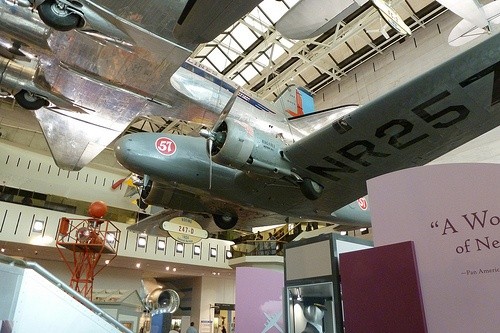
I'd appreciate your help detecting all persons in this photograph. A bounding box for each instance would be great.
[217,325,222,333]
[222,326,226,333]
[237,228,284,255]
[171,325,179,333]
[186,321,197,333]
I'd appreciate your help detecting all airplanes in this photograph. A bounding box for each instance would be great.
[0,0,499,230]
